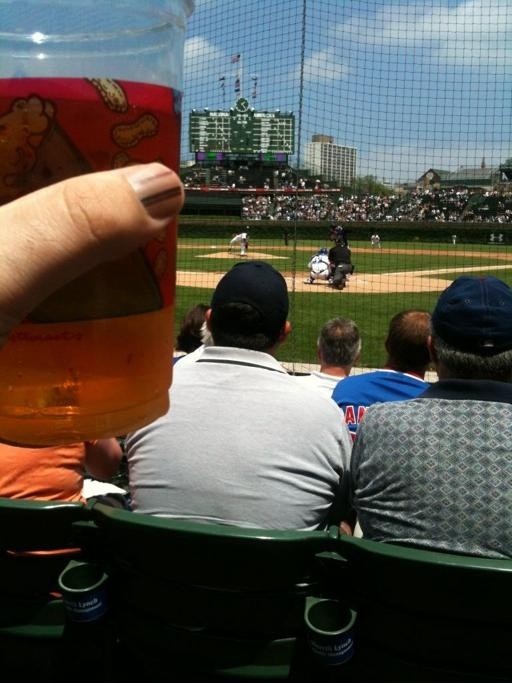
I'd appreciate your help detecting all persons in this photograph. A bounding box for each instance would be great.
[0,435,126,630]
[120,260,357,660]
[328,237,354,290]
[370,231,381,248]
[301,315,362,401]
[351,273,510,558]
[169,302,212,367]
[1,159,188,331]
[327,307,437,437]
[301,246,333,284]
[227,229,250,256]
[328,225,349,249]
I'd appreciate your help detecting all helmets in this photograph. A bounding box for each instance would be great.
[318,246,330,254]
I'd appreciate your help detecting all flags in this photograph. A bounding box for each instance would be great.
[230,53,241,63]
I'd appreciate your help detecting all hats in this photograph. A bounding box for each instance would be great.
[210,257,290,334]
[432,272,511,356]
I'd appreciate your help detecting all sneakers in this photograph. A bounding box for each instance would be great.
[303,278,346,290]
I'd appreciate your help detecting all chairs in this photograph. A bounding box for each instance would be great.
[89,501,337,683]
[331,533,512,682]
[1,496,109,683]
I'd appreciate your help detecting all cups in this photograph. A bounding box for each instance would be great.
[0,0,200,446]
